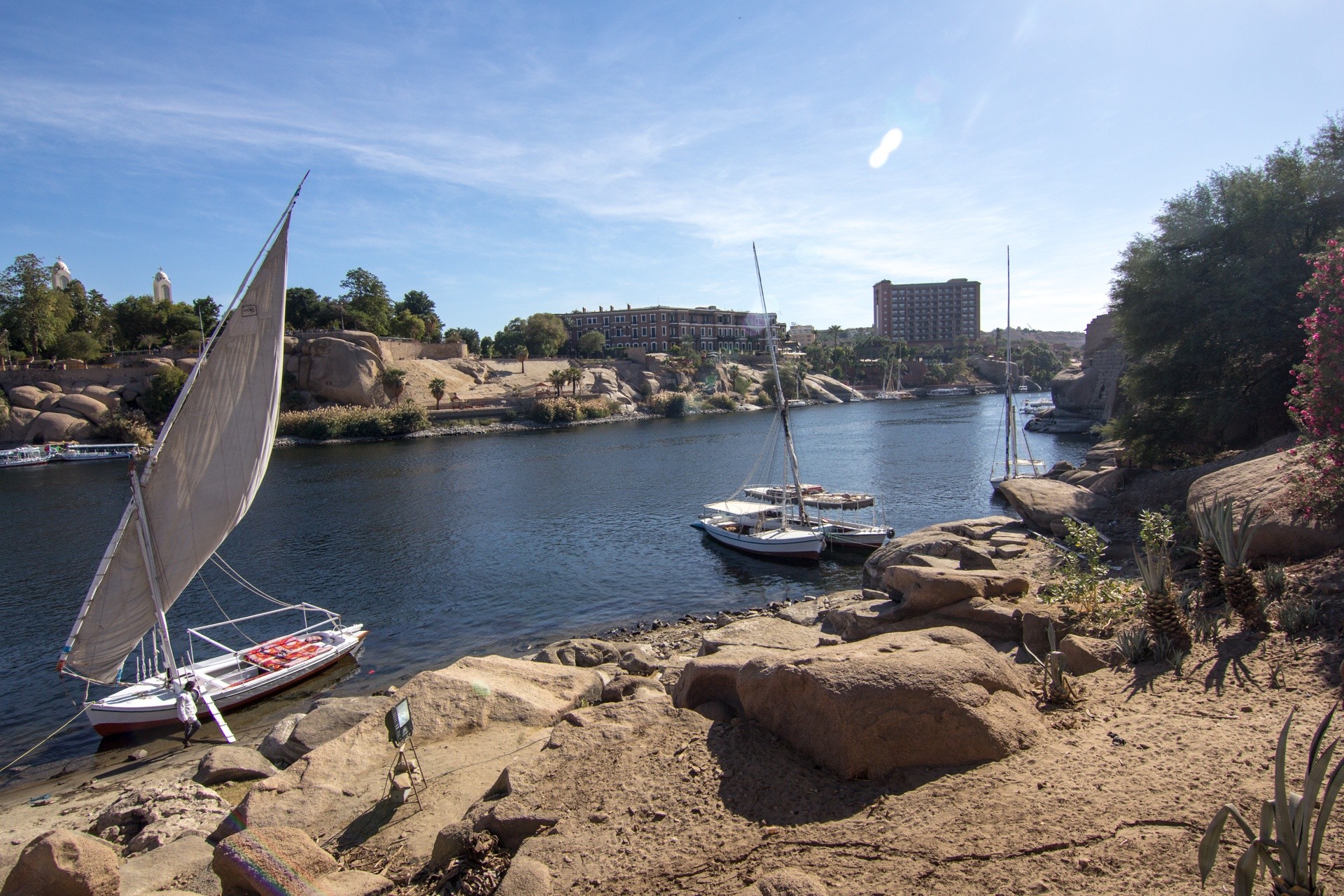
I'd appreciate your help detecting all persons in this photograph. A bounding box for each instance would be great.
[176,681,202,748]
[44,445,49,456]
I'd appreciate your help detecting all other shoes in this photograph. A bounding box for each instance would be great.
[182,740,193,748]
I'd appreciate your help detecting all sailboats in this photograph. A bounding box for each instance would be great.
[988,245,1048,494]
[52,170,369,747]
[693,242,830,568]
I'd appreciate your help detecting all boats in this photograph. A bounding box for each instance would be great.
[0,440,143,468]
[926,387,968,396]
[1019,398,1055,414]
[744,481,895,553]
